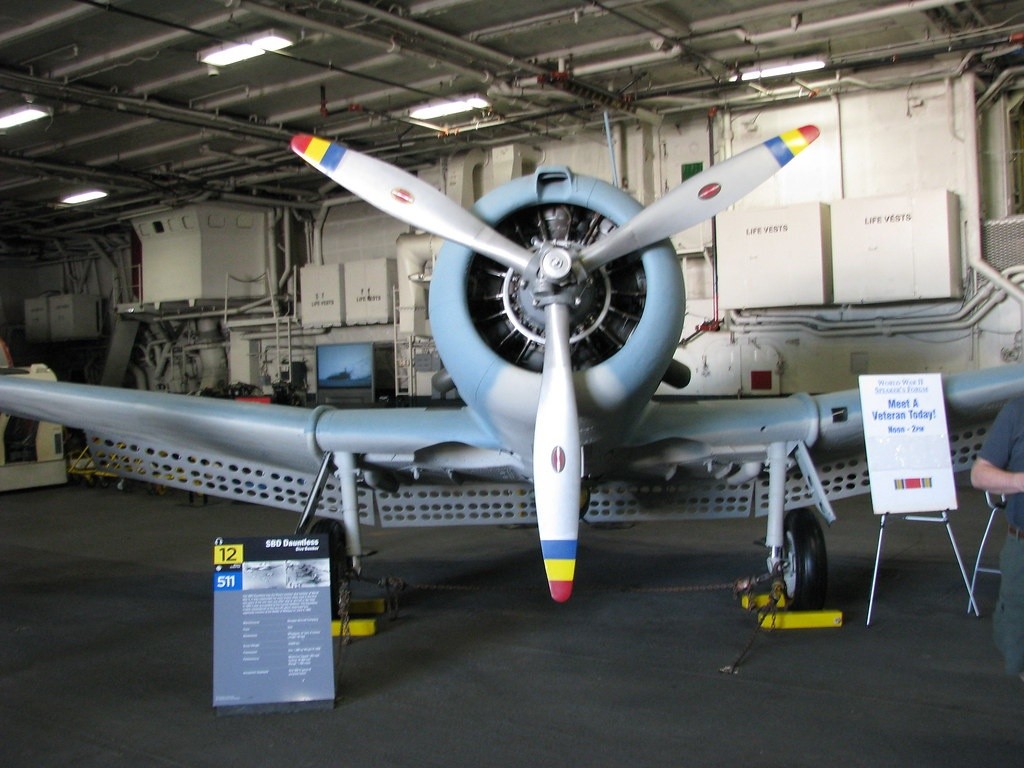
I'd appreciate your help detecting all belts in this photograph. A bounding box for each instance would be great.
[1006,523,1024,542]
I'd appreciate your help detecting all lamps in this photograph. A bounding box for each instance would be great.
[407,91,492,120]
[725,54,828,83]
[0,102,52,130]
[196,27,298,65]
[61,188,107,205]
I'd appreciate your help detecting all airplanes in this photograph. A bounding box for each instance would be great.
[1,132,1022,619]
